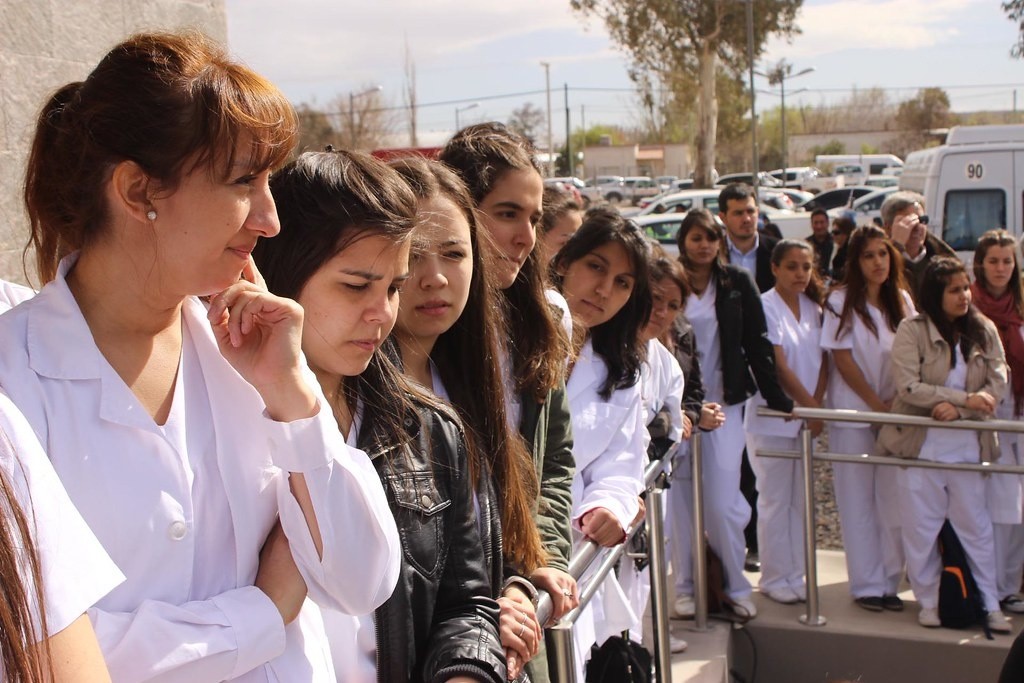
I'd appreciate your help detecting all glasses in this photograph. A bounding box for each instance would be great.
[919,215,929,225]
[831,228,843,235]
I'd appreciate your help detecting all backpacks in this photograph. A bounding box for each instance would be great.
[935,545,994,641]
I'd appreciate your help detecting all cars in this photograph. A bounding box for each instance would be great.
[632,166,902,264]
[544,175,696,202]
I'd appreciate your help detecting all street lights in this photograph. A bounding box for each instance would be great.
[455,102,481,133]
[748,68,814,168]
[541,61,555,177]
[349,86,385,150]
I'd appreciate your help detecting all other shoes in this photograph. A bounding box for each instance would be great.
[741,551,761,572]
[860,596,884,612]
[883,596,904,611]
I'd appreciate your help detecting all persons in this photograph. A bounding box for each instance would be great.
[0,33,403,683]
[0,393,126,683]
[253,122,1024,683]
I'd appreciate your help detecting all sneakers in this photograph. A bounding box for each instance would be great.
[919,607,941,627]
[1002,592,1024,613]
[673,593,697,617]
[987,610,1012,633]
[733,598,758,618]
[669,634,687,653]
[766,585,808,604]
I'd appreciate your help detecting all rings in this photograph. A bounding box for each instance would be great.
[521,610,529,625]
[561,587,574,600]
[518,624,527,637]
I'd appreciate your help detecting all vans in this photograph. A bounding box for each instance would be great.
[815,154,904,175]
[901,125,1024,282]
